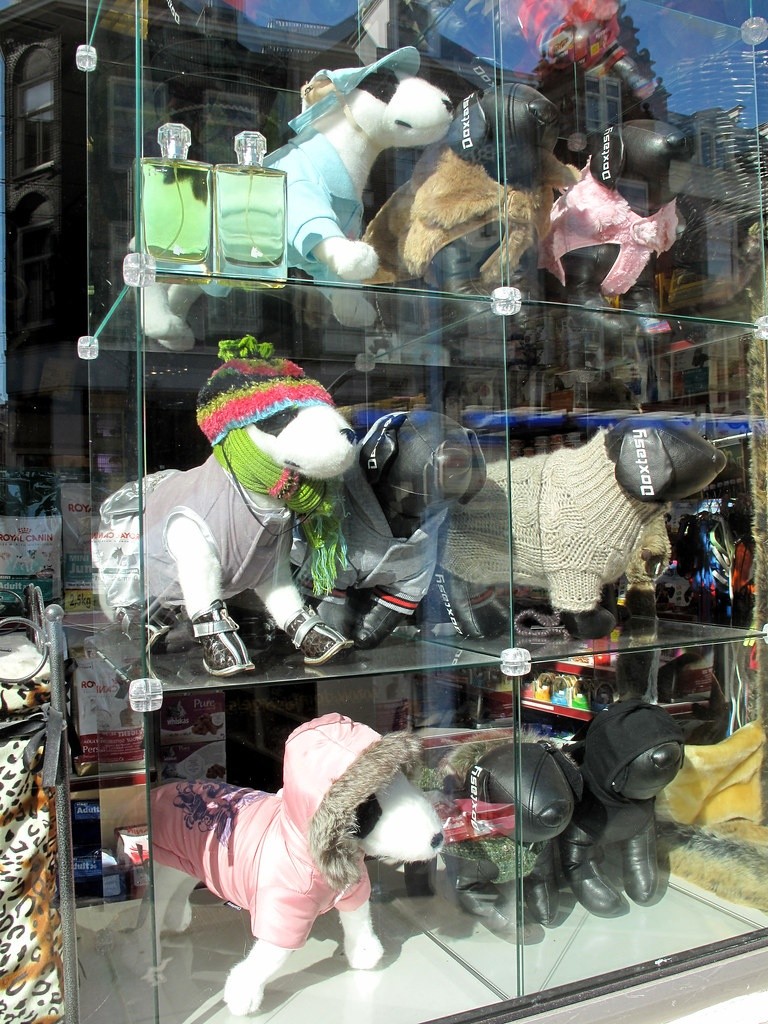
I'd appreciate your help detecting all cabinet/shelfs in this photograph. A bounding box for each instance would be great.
[59,0,768,1024]
[433,294,747,723]
[226,675,419,763]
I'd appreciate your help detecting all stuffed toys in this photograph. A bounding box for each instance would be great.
[122,713,445,1016]
[406,727,583,944]
[128,47,452,351]
[537,697,686,915]
[293,79,564,356]
[232,409,487,647]
[531,120,693,351]
[416,418,727,641]
[87,336,360,677]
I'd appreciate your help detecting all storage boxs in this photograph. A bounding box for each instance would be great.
[153,691,226,747]
[154,739,227,784]
